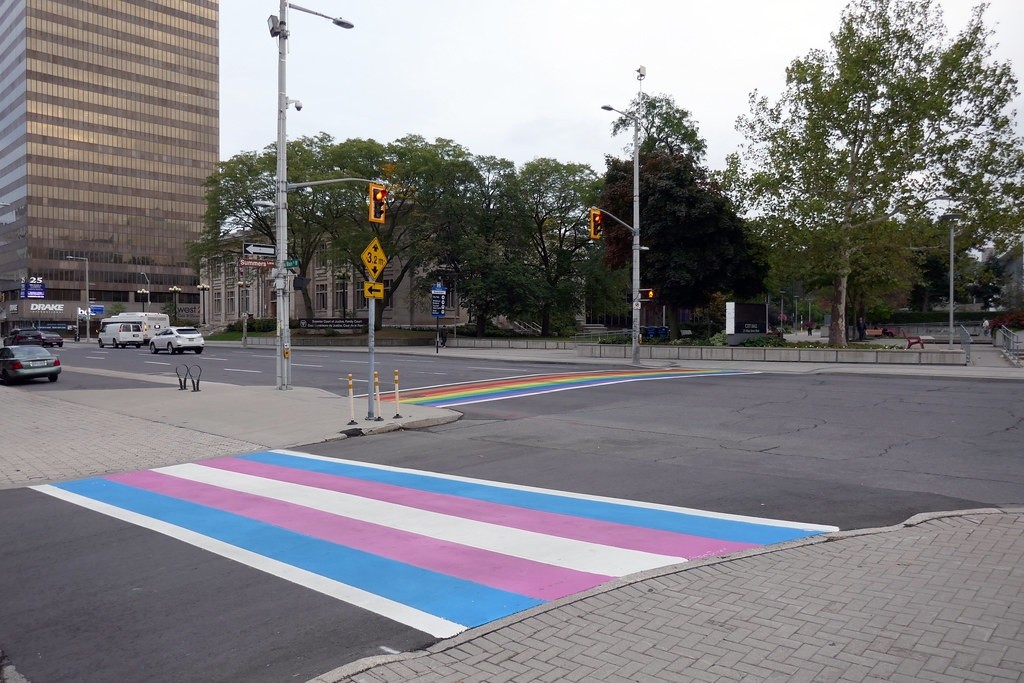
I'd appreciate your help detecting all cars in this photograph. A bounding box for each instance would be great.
[0,345,62,386]
[149,326,206,355]
[2,327,43,346]
[36,330,64,347]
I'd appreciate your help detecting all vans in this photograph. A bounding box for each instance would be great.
[98,322,145,349]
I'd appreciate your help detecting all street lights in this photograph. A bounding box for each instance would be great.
[779,290,786,332]
[266,0,355,394]
[335,267,352,329]
[197,283,211,324]
[794,296,800,335]
[66,255,91,343]
[238,281,251,331]
[943,212,963,351]
[169,285,181,325]
[807,298,813,333]
[601,104,642,366]
[137,289,150,312]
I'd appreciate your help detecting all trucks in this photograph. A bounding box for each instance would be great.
[99,311,171,345]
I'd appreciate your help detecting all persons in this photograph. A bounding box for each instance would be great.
[857,317,867,342]
[806,320,813,336]
[440,325,448,348]
[982,317,990,337]
[880,327,893,336]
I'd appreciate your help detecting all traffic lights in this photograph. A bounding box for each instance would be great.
[369,182,388,224]
[590,208,604,240]
[646,290,654,299]
